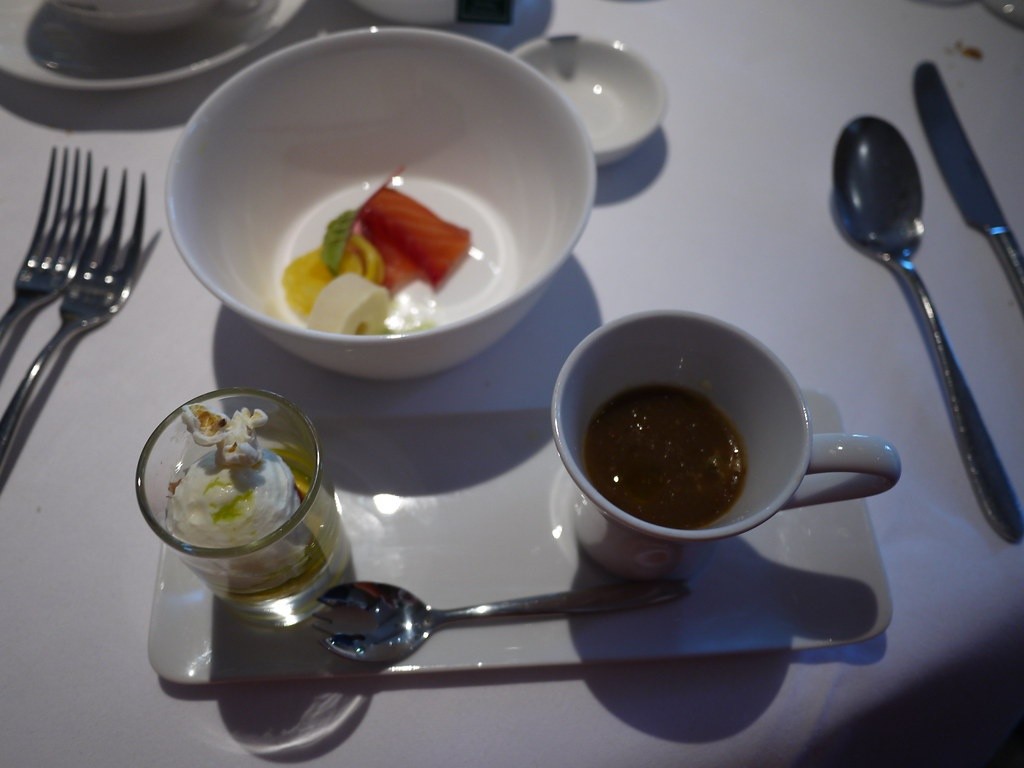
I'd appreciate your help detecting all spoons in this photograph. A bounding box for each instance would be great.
[313,581,687,665]
[831,116,1024,543]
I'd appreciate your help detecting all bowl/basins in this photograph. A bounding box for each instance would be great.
[165,26,595,379]
[48,0,212,35]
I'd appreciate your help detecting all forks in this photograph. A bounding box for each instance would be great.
[0,147,91,359]
[0,167,146,477]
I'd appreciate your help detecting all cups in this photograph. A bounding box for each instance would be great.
[550,309,902,542]
[137,387,345,627]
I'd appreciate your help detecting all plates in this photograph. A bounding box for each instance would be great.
[148,392,894,684]
[513,33,670,166]
[0,0,305,89]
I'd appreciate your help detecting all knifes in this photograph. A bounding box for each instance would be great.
[914,62,1024,318]
